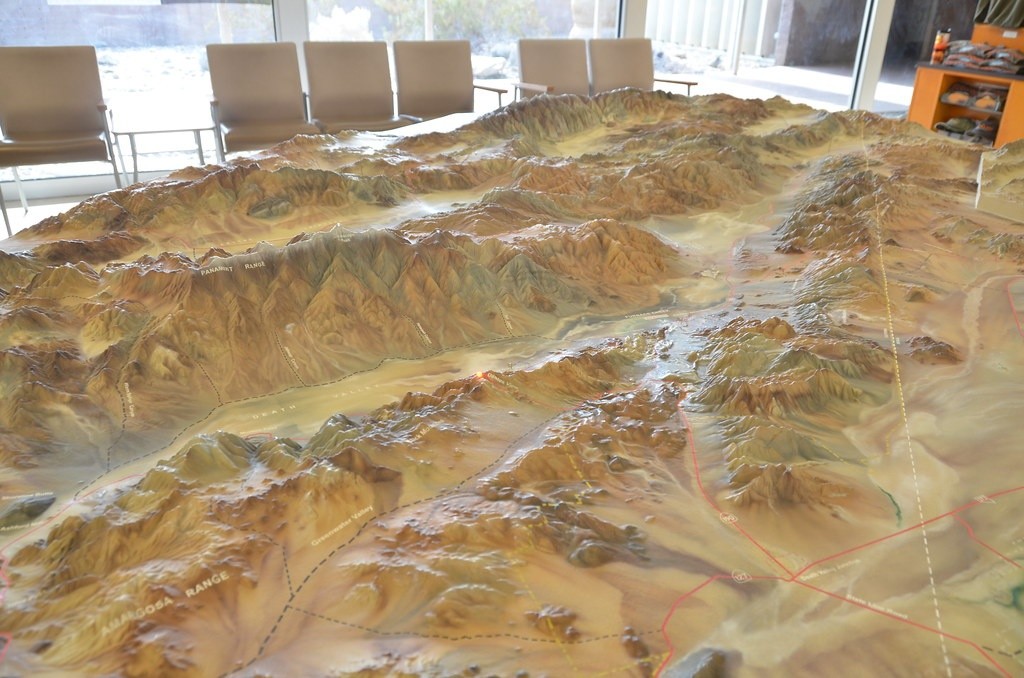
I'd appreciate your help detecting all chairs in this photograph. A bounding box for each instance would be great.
[0,45,121,237]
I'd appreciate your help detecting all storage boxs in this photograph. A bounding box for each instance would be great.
[971,23,1024,51]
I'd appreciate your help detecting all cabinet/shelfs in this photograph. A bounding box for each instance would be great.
[907,61,1024,150]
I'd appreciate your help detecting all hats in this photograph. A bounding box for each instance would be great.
[934,117,975,133]
[967,119,998,141]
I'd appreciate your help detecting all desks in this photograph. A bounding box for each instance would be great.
[114,122,211,184]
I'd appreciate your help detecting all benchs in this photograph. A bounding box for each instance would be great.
[512,39,698,94]
[205,42,507,161]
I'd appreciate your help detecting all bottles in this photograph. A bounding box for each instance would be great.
[930,27,951,65]
